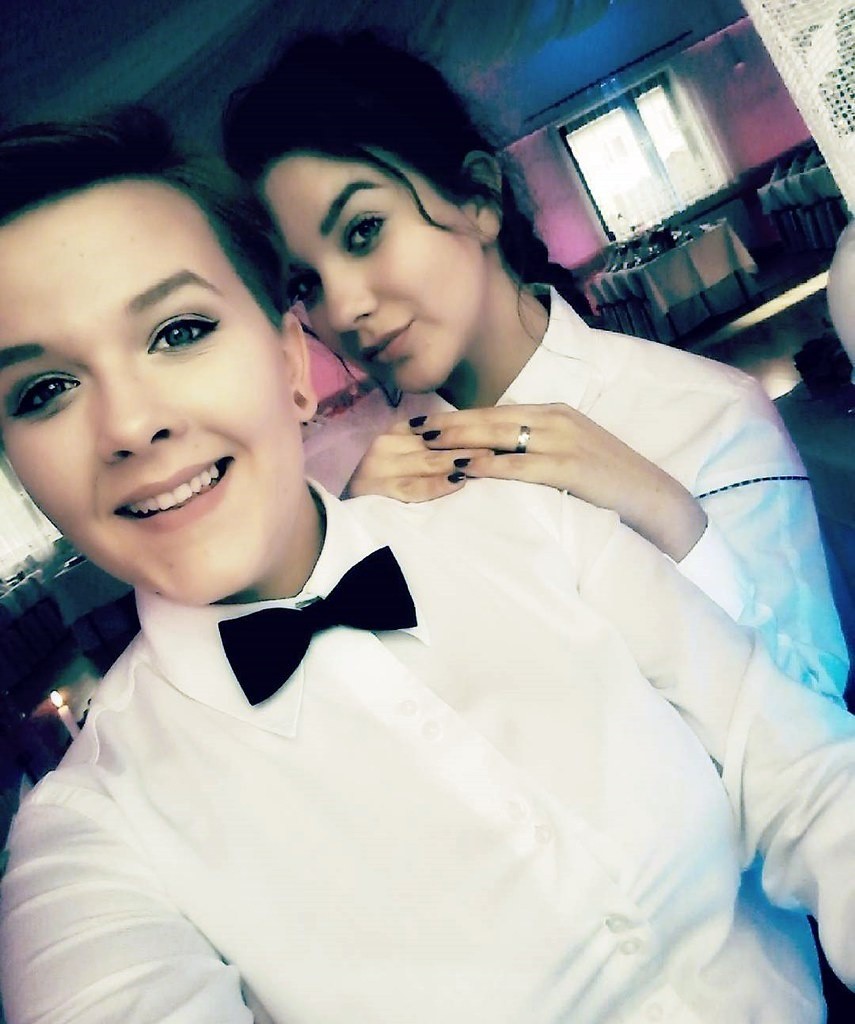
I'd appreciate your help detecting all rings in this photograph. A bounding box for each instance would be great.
[517,425,531,451]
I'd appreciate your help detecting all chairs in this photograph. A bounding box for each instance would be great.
[587,271,662,344]
[756,168,847,251]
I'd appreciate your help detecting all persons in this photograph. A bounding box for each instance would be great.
[0,122,855,1024]
[223,28,849,703]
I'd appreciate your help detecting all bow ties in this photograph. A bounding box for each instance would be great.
[218,546,419,707]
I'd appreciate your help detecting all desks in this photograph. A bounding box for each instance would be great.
[0,569,61,664]
[51,564,135,651]
[583,219,760,342]
